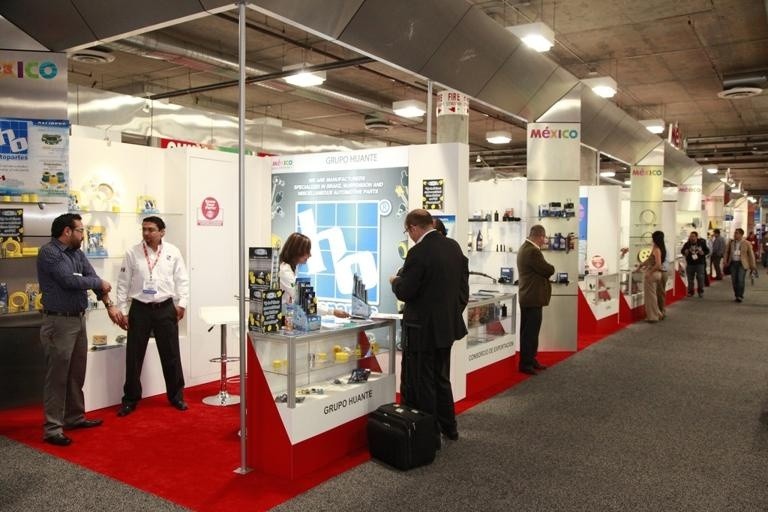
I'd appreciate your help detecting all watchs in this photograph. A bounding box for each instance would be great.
[105,300,112,306]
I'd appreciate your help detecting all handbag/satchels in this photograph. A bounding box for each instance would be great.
[723,266,731,275]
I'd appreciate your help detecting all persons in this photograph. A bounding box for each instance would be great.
[38,213,124,448]
[680,228,768,303]
[635,231,669,316]
[632,232,666,323]
[390,208,471,451]
[517,225,554,375]
[278,233,351,318]
[116,216,190,416]
[431,220,446,238]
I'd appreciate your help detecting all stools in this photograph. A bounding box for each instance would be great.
[198,302,243,408]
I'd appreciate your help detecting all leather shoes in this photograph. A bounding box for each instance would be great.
[46,400,187,445]
[519,363,548,376]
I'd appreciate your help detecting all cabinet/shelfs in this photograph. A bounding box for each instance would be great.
[621,268,649,322]
[578,270,619,335]
[470,217,524,286]
[0,200,73,322]
[72,207,189,352]
[468,289,518,393]
[242,316,399,476]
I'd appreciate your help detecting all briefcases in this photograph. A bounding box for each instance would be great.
[367,403,437,471]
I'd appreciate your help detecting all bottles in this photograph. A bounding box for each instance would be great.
[475,229,483,252]
[494,210,498,221]
[502,304,507,317]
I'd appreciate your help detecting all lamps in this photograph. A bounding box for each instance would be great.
[278,40,327,86]
[637,103,666,134]
[578,57,620,99]
[250,102,282,130]
[388,79,429,120]
[142,99,150,113]
[484,115,514,144]
[498,0,556,52]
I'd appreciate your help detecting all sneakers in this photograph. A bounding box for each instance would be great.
[687,292,743,302]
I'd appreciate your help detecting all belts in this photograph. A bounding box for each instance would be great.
[44,310,85,318]
[132,297,172,311]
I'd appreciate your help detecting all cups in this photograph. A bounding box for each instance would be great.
[20,193,29,202]
[273,360,282,369]
[29,193,38,202]
[3,195,11,202]
[113,206,120,213]
[42,172,65,185]
[81,206,88,211]
[315,352,349,363]
